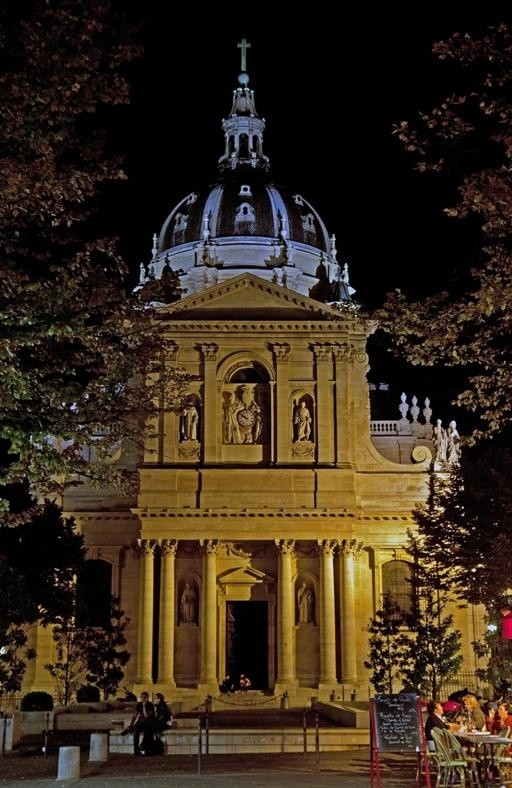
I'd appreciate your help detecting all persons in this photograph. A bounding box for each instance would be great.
[425,700,473,753]
[122,691,155,755]
[223,675,236,696]
[180,400,200,442]
[239,673,252,695]
[458,695,486,732]
[293,401,313,444]
[297,580,313,623]
[484,701,512,779]
[178,581,198,624]
[137,692,174,749]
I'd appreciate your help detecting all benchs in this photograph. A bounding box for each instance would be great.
[110,716,202,736]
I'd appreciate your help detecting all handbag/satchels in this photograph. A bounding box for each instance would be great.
[145,735,164,757]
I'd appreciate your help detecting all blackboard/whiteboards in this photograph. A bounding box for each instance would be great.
[370,693,427,752]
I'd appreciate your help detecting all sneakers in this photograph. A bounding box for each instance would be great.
[121,729,129,736]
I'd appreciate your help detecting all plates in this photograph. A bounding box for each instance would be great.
[472,731,491,736]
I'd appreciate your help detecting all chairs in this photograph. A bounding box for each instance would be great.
[415,718,512,788]
